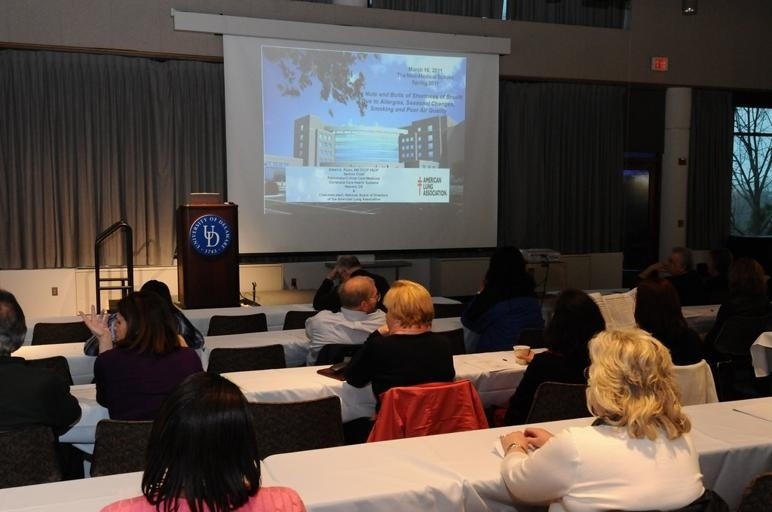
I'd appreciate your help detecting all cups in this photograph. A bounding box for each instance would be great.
[512,344,530,367]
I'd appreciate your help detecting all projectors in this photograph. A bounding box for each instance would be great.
[520,248,561,264]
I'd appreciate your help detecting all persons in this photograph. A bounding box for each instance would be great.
[499,328,708,511]
[77,280,206,421]
[313,255,389,314]
[461,247,544,354]
[486,287,606,428]
[633,247,772,402]
[1,290,81,488]
[305,275,388,366]
[98,371,311,512]
[346,280,455,413]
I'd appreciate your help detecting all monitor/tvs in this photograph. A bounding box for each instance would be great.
[188,191,221,205]
